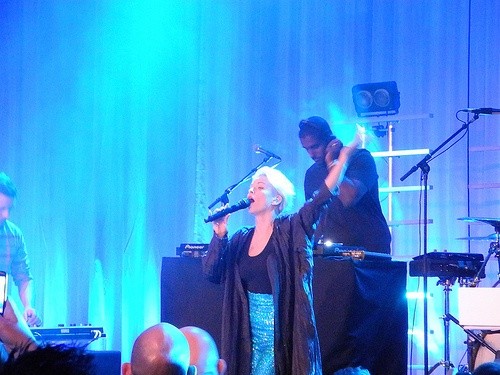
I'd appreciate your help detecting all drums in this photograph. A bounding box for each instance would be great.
[470,332,500,371]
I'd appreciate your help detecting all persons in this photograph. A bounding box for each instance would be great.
[298,116,392,260]
[473,362,500,375]
[202,124,361,375]
[123,322,227,375]
[1,172,41,328]
[0,298,95,375]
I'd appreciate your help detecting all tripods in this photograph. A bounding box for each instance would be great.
[427,279,500,375]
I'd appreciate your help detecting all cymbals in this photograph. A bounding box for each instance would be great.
[454,232,500,242]
[455,217,500,226]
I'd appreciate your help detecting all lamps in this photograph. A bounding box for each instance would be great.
[351,81,400,117]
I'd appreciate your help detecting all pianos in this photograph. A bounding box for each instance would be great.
[29,323,106,350]
[409,251,486,375]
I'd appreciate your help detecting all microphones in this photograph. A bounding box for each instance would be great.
[204,198,251,223]
[253,144,281,160]
[460,108,500,115]
